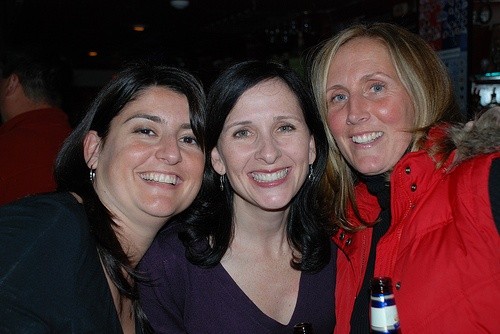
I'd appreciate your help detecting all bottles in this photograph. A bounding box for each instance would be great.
[262,7,313,49]
[369,277,402,334]
[292,322,314,334]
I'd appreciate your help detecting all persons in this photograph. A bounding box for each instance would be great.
[0,64,208,334]
[0,52,74,206]
[307,21,500,333]
[134,58,337,334]
